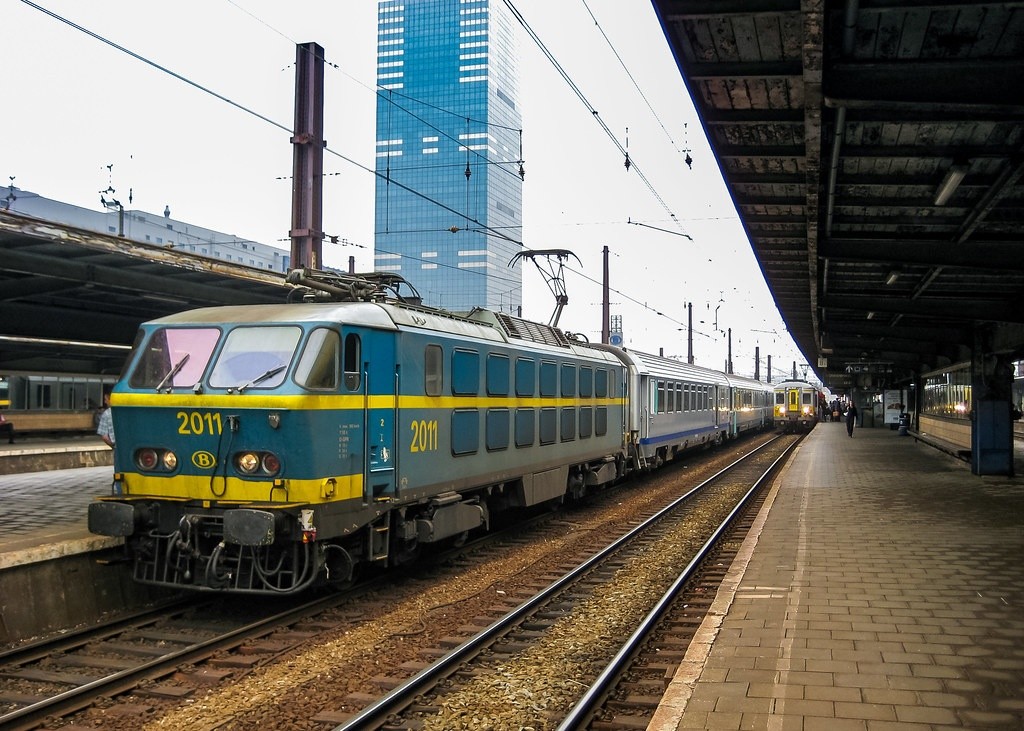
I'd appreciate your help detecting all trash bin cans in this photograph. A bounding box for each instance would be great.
[898,413,911,436]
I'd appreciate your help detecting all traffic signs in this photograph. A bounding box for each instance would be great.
[844,361,896,374]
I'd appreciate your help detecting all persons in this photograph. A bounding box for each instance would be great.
[842,400,859,440]
[821,400,847,422]
[96,389,123,496]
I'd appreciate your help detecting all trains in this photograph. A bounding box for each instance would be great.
[86,265,778,596]
[920,369,971,417]
[773,379,825,433]
[0,370,122,434]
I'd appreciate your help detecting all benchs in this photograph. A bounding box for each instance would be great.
[905,428,972,464]
